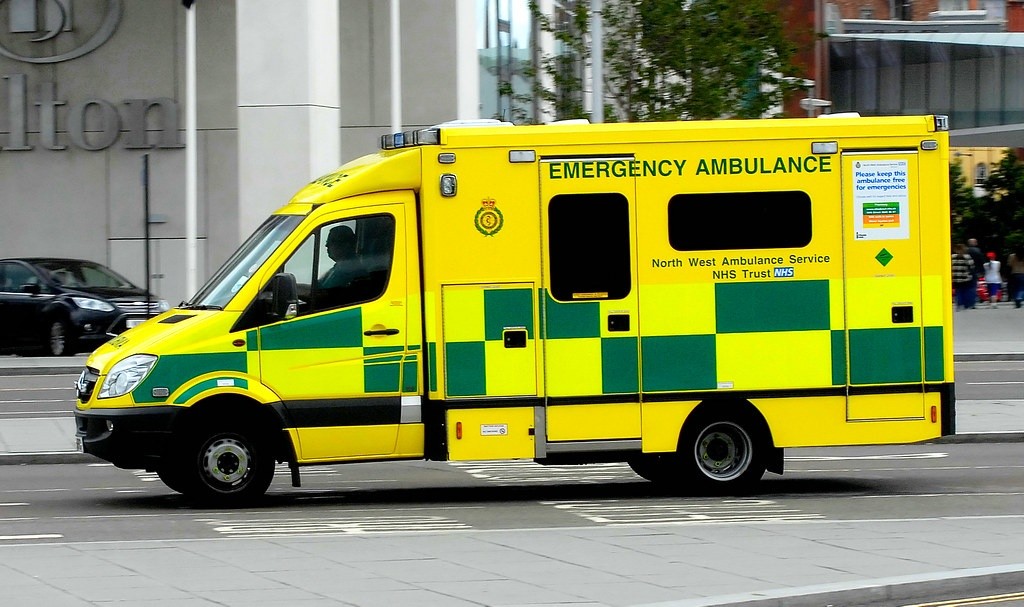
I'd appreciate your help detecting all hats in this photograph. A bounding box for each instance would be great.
[987,252,997,260]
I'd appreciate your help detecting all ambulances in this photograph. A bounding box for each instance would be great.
[72,111,957,512]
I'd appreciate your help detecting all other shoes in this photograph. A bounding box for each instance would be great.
[991,302,998,309]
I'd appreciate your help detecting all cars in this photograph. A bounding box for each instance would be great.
[0,258,171,358]
[976,282,1002,303]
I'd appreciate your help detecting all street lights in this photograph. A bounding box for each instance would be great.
[800,98,831,114]
[783,77,814,118]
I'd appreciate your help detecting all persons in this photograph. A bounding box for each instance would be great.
[951,238,1024,312]
[297,225,371,310]
